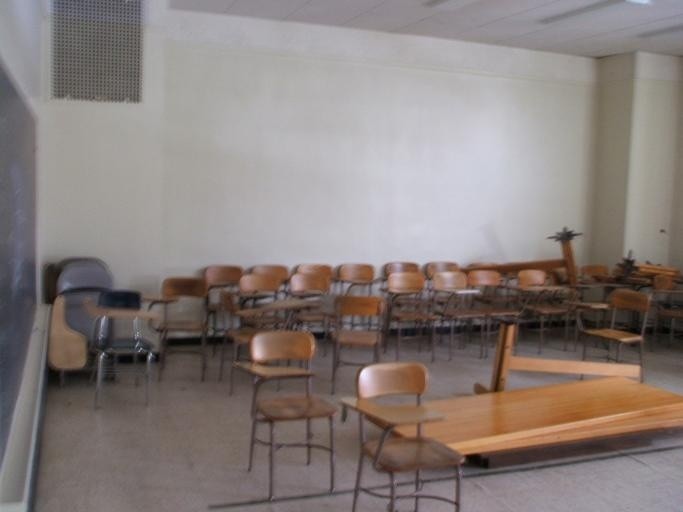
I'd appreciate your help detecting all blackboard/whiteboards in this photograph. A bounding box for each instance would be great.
[0,61,36,466]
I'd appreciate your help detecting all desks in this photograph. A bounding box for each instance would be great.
[367,376,682,469]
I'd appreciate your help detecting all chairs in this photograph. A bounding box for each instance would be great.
[353,361,466,511]
[52,259,681,396]
[247,330,338,501]
[94,288,154,409]
